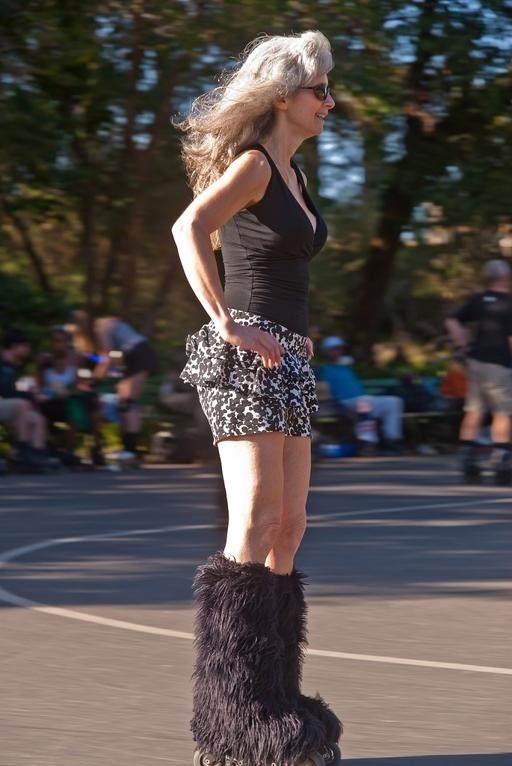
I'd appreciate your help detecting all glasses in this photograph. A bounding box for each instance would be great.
[300,83,333,101]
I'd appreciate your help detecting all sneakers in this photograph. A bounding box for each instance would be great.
[7,445,196,474]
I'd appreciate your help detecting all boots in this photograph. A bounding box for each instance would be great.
[456,439,482,485]
[190,550,327,766]
[489,442,511,485]
[279,565,341,766]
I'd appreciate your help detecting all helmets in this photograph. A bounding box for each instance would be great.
[320,336,345,354]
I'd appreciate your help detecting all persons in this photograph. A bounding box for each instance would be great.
[173,28,342,762]
[311,326,491,455]
[2,306,218,473]
[445,258,511,469]
